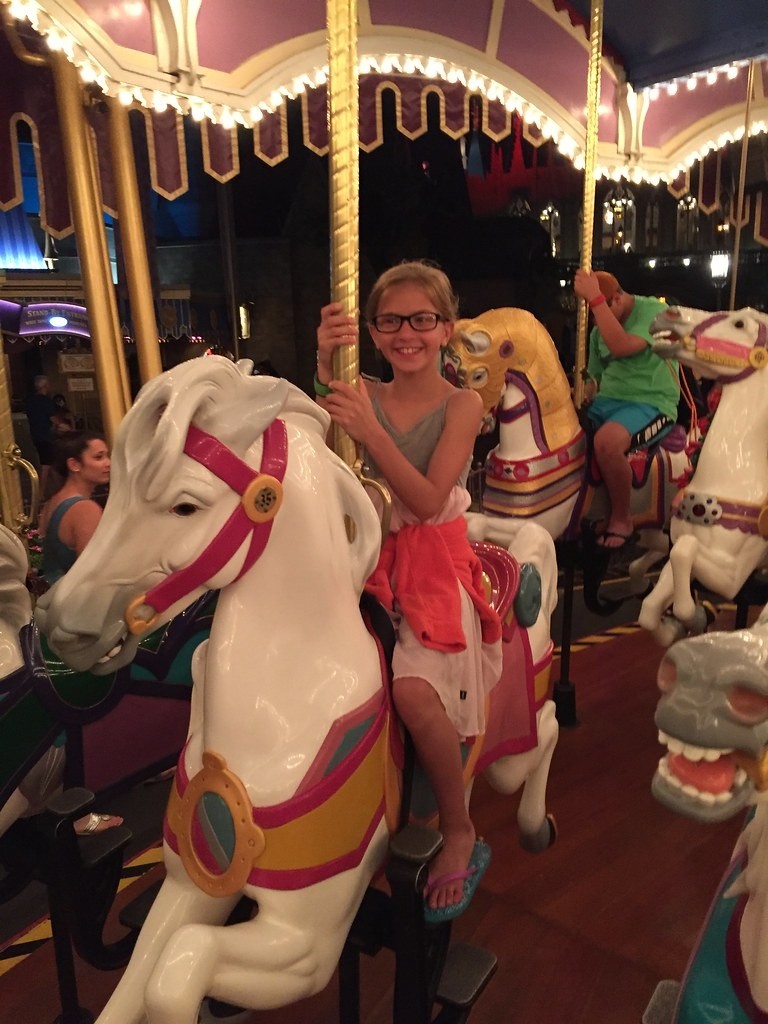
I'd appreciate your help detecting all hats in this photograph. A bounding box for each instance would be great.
[592,271,620,297]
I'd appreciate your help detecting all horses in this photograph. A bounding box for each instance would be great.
[0,303,768,1024]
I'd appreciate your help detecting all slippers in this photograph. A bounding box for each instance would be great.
[420,840,492,922]
[595,530,641,551]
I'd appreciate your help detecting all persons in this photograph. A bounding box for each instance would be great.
[51,393,75,434]
[39,432,109,585]
[573,267,682,554]
[311,263,492,923]
[22,376,54,504]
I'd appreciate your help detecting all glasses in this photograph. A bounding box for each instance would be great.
[369,311,447,334]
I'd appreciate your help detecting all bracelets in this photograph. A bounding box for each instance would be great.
[589,295,604,308]
[312,370,333,397]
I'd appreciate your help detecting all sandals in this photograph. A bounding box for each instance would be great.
[76,813,123,836]
